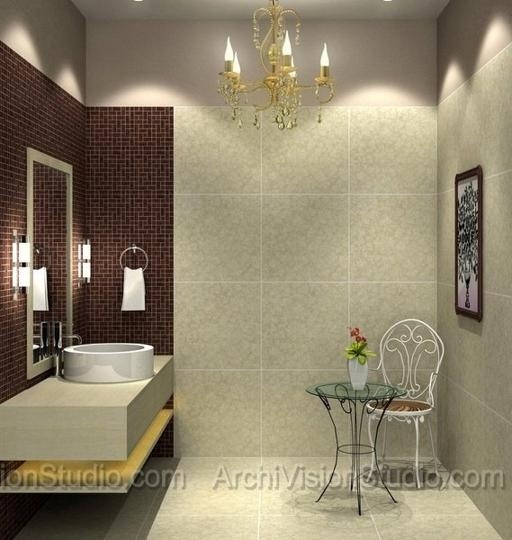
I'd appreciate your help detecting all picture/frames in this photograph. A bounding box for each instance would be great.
[454,166,483,323]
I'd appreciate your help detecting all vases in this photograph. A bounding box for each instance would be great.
[348,359,368,390]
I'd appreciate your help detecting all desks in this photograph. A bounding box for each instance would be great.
[306,382,407,516]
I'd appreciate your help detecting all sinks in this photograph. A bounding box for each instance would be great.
[32,344,40,364]
[63,342,155,384]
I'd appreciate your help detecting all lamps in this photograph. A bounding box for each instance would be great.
[219,0,334,131]
[18,233,31,294]
[82,236,91,285]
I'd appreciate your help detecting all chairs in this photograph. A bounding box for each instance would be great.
[367,318,445,489]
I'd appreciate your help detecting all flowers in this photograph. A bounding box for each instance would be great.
[345,328,377,364]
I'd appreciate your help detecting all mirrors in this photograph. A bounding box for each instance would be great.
[26,146,74,380]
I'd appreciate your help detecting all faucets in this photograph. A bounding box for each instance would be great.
[51,320,83,377]
[33,321,50,360]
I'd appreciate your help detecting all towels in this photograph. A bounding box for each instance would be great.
[33,266,49,312]
[120,267,146,312]
